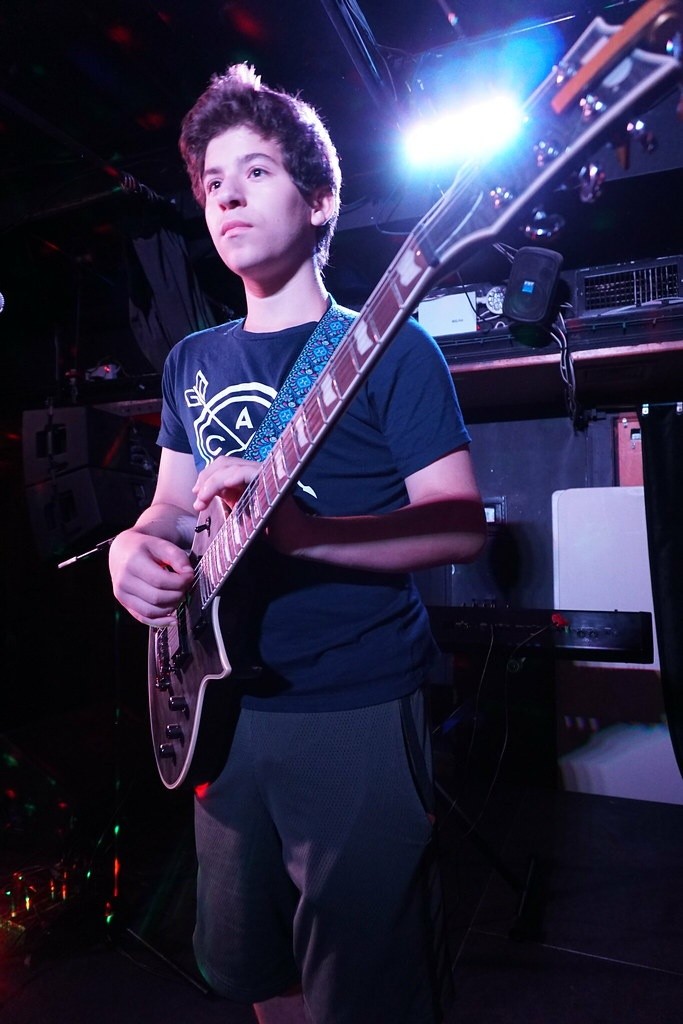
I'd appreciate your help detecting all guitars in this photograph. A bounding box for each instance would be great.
[145,0,683,792]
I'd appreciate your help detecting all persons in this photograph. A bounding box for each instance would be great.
[109,61,486,1024]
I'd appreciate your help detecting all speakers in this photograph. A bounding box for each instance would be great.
[21,407,163,562]
[501,246,564,326]
